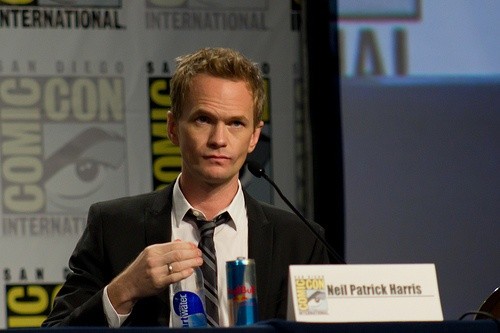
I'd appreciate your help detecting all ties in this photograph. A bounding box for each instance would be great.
[184,212,232,329]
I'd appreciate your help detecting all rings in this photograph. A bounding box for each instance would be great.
[166,263,176,275]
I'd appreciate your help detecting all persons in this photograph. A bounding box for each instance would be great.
[41,46,330,329]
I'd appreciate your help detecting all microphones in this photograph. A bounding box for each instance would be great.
[248,160,347,264]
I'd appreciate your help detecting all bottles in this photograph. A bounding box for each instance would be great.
[169,266,208,329]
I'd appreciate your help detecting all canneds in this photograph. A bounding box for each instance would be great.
[225,257,259,327]
